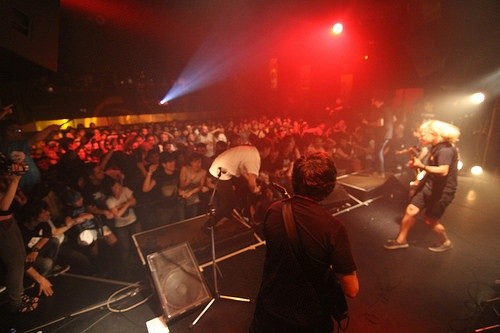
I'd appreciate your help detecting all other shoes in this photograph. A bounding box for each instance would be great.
[372,171,385,179]
[21,281,36,290]
[0,286,7,292]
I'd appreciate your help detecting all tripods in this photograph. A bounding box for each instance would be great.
[189,171,255,329]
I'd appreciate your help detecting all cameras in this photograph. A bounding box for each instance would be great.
[8,164,29,174]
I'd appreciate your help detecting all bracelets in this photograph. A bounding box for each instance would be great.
[424,165,426,169]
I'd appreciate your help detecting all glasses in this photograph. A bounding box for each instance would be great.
[95,169,103,175]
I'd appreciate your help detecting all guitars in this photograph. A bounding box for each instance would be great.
[269,181,349,319]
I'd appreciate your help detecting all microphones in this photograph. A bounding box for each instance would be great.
[219,167,227,174]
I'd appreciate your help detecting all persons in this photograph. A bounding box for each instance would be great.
[250,151,359,333]
[0,94,462,313]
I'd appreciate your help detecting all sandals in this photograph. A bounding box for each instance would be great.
[8,295,40,314]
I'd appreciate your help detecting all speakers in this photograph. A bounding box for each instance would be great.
[318,183,351,211]
[339,174,388,200]
[147,241,214,324]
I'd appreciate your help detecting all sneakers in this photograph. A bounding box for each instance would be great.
[384,240,409,250]
[46,264,70,277]
[428,242,453,252]
